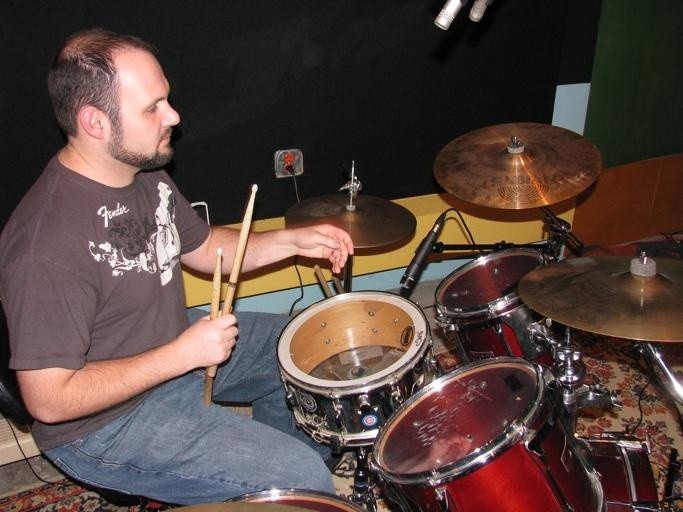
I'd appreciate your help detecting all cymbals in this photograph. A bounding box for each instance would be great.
[165,502,319,511]
[285,194,415,248]
[434,122,602,209]
[518,256,682,344]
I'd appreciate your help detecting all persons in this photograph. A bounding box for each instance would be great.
[0,28,354,506]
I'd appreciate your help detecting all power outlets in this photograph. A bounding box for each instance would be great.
[274,150,303,178]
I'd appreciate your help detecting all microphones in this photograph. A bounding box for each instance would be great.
[400,212,448,289]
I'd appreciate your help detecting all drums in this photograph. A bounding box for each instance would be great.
[575,432,660,512]
[218,488,371,512]
[433,249,563,367]
[276,292,433,450]
[365,357,607,511]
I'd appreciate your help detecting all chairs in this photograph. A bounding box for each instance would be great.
[1,308,181,505]
